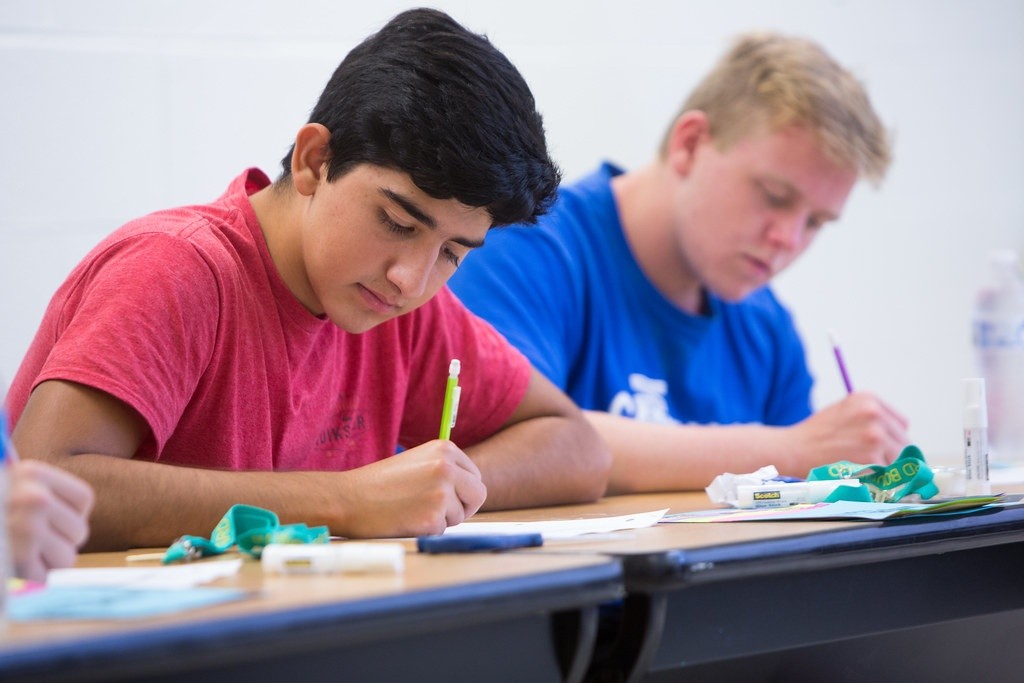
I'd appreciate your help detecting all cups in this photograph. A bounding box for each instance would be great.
[938,376,989,488]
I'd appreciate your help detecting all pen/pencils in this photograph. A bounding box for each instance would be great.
[832,346,856,394]
[438,357,461,440]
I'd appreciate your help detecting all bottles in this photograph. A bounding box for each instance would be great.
[969,249,1024,483]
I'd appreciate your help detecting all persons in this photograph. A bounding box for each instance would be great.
[0,410,95,584]
[5,8,612,551]
[447,33,910,496]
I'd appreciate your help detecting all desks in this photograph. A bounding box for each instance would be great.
[435,471,1024,683]
[0,530,622,683]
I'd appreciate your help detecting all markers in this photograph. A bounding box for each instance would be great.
[960,376,990,497]
[737,477,860,510]
